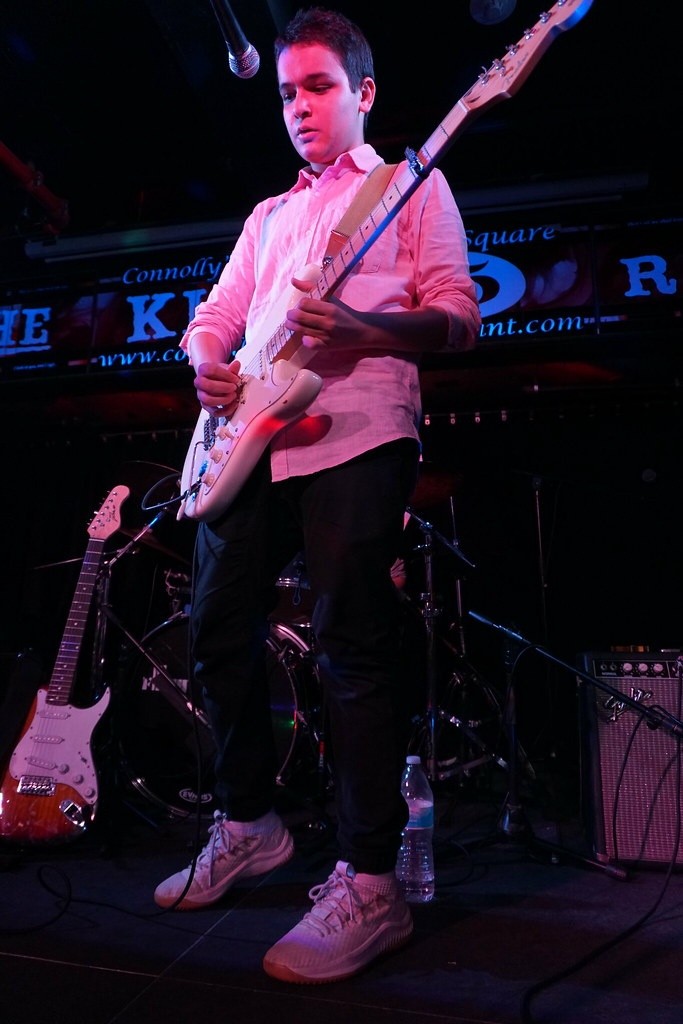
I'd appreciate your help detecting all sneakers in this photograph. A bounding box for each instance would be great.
[263,861,414,983]
[153,811,295,909]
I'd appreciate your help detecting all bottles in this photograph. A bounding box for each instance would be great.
[395,756,434,903]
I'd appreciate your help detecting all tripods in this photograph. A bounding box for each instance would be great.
[399,494,629,882]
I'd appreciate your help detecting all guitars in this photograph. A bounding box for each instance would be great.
[177,0,597,524]
[0,485,131,842]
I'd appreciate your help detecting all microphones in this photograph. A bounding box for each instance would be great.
[210,0,261,79]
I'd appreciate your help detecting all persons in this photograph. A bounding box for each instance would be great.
[151,9,480,983]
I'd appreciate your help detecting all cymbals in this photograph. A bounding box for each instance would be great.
[118,528,194,568]
[406,461,459,515]
[118,458,182,516]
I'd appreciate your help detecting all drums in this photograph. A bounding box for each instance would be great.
[117,609,323,823]
[275,549,318,592]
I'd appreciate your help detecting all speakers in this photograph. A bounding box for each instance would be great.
[578,646,683,872]
